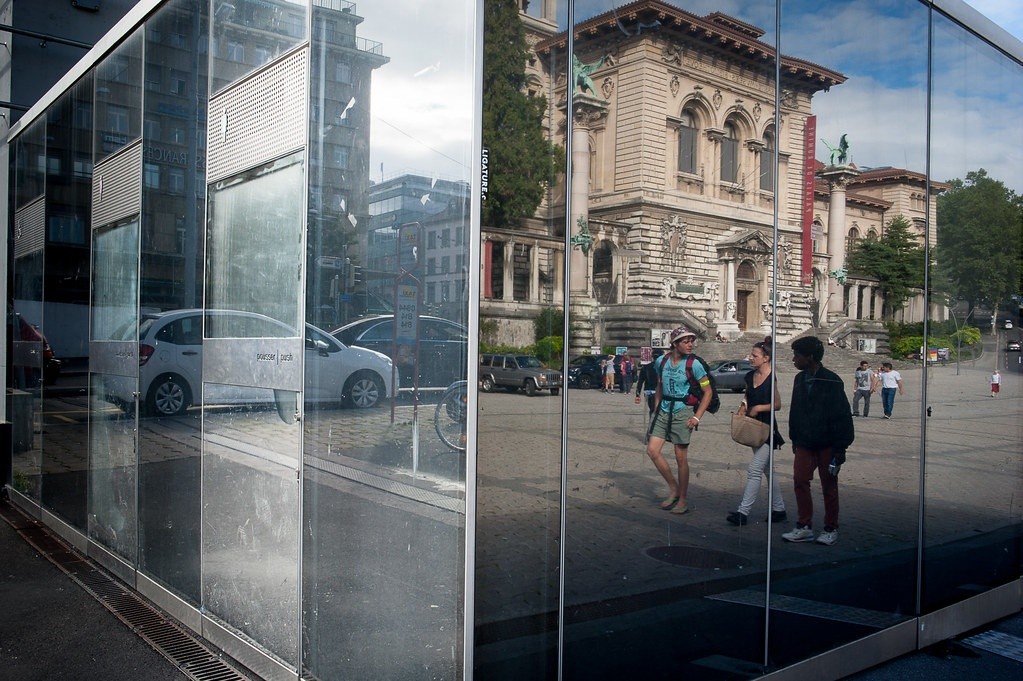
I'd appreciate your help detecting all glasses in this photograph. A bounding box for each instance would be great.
[757,343,768,355]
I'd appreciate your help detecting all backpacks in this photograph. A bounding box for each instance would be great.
[659,352,720,414]
[625,360,631,374]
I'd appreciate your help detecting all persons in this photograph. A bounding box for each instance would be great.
[874,363,903,419]
[646,327,712,515]
[828,337,836,347]
[601,351,635,395]
[782,335,854,544]
[989,370,1001,397]
[852,360,876,418]
[727,336,786,523]
[716,331,728,342]
[635,348,665,445]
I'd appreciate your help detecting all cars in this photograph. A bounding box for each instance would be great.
[327,313,469,389]
[7,312,63,386]
[560,353,639,388]
[1006,340,1020,352]
[1003,320,1013,329]
[98,307,401,417]
[706,360,757,393]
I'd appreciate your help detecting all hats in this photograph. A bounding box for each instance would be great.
[670,327,697,343]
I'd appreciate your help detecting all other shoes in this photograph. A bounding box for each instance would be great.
[852,413,859,417]
[863,414,867,418]
[727,512,747,525]
[765,511,788,523]
[884,414,890,419]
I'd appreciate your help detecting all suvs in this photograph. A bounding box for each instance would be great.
[479,353,563,396]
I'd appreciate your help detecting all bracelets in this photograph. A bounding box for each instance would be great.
[693,416,699,422]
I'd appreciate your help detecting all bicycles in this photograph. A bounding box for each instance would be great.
[434,380,467,452]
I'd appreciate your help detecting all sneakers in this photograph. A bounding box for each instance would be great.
[781,525,814,542]
[816,528,841,545]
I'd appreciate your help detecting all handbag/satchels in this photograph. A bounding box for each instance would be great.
[730,406,770,449]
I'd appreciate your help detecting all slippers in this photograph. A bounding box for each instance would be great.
[662,497,679,509]
[671,508,688,514]
